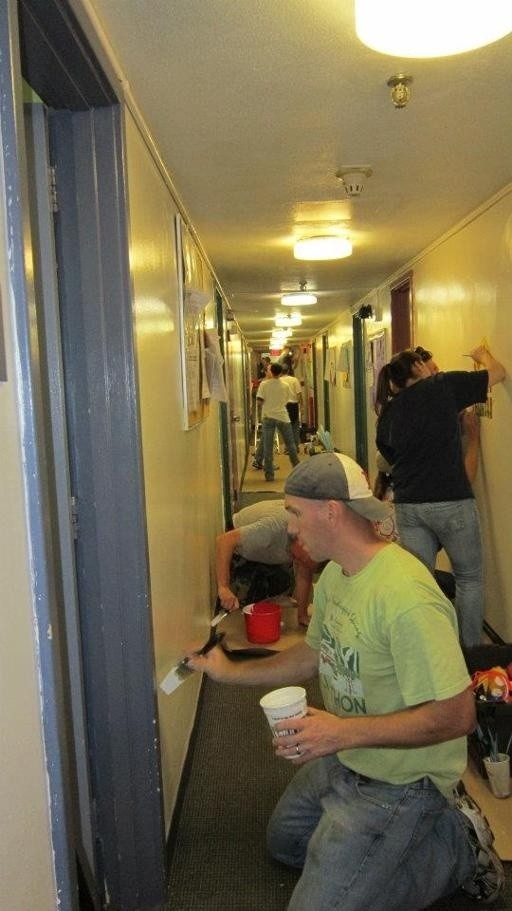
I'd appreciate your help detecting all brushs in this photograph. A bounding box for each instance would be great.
[159,631,226,696]
[210,609,230,627]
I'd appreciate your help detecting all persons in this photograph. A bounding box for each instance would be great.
[217,499,319,629]
[179,451,505,909]
[250,352,302,481]
[377,342,510,656]
[373,342,482,561]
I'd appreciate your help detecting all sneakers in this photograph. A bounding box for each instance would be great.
[253,461,265,469]
[456,795,505,904]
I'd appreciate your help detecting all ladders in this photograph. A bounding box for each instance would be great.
[254,396,280,455]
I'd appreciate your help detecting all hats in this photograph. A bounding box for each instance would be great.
[284,453,395,522]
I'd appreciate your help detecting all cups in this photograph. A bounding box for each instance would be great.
[482,754,510,798]
[259,685,313,761]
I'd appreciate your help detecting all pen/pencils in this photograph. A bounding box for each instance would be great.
[462,354,473,357]
[477,724,512,763]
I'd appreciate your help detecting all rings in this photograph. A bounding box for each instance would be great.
[296,745,299,756]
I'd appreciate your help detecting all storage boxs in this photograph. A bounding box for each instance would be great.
[461,641,512,779]
